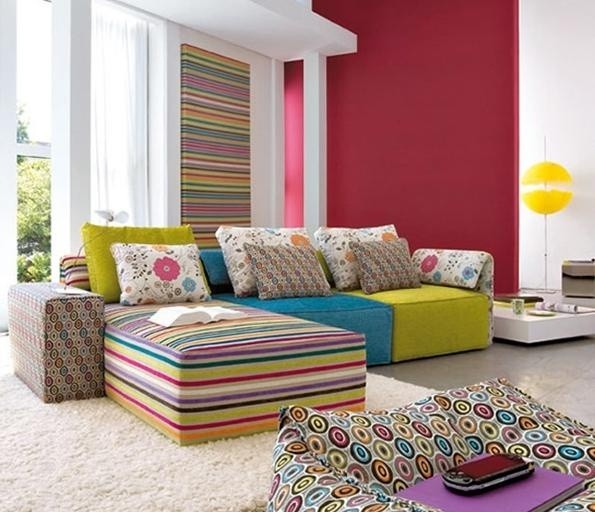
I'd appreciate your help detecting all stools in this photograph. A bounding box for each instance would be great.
[267,371,595,512]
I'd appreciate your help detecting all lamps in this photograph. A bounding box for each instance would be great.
[520,133,575,296]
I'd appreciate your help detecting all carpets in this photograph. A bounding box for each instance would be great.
[2,337,447,511]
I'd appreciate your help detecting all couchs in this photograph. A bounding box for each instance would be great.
[11,223,493,446]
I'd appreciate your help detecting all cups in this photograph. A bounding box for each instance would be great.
[512,298,525,319]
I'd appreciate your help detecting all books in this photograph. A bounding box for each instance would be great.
[535,301,595,314]
[394,450,587,512]
[147,305,248,327]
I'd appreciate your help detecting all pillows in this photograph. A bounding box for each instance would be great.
[243,242,333,297]
[215,225,334,298]
[349,238,421,295]
[314,224,399,292]
[111,241,213,302]
[83,224,215,303]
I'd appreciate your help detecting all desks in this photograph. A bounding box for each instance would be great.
[562,259,595,299]
